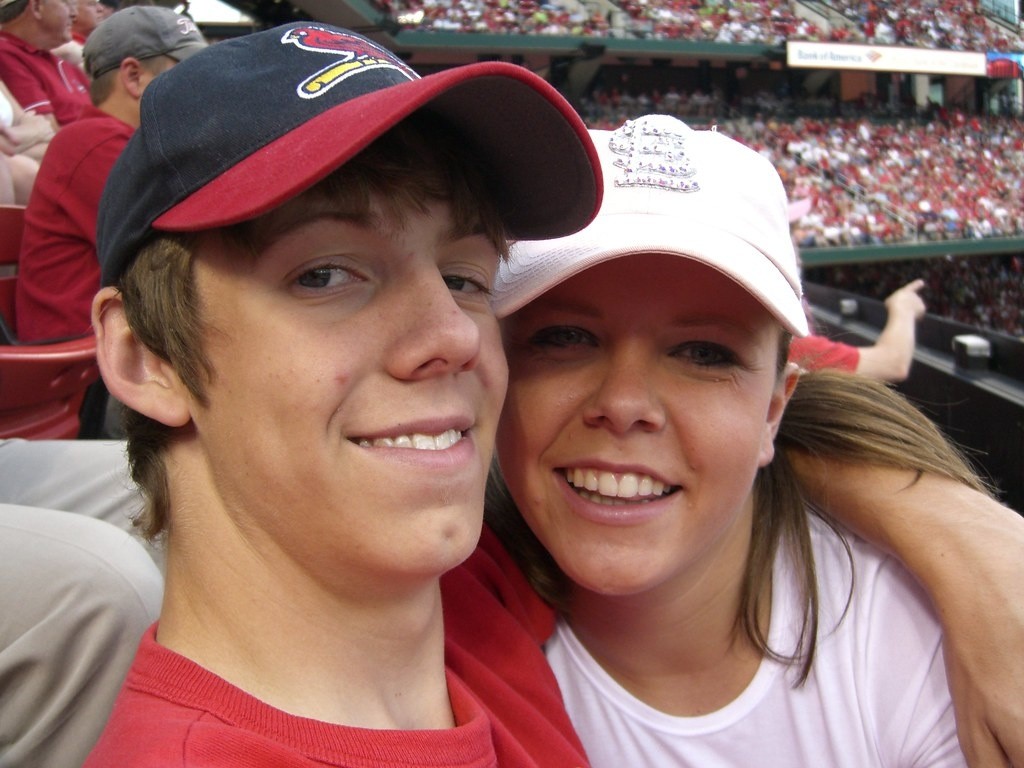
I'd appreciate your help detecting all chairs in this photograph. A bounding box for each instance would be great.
[0,207,99,440]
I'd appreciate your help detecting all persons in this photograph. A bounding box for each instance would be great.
[0,0,1024,768]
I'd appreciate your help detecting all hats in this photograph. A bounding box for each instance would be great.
[93,22,605,293]
[83,7,207,75]
[491,112,809,340]
